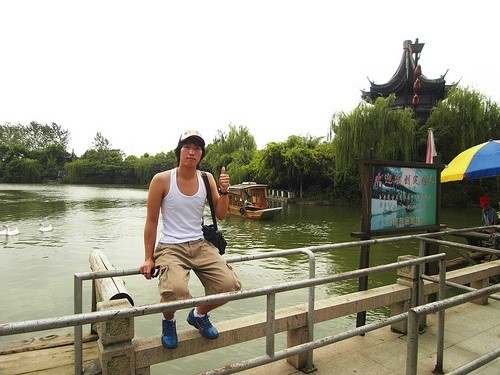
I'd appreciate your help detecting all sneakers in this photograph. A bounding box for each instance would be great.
[186,308,219,340]
[161,319,178,350]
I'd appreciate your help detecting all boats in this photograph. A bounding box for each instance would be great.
[226,181,283,221]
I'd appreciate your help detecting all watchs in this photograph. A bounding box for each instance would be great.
[218,187,228,195]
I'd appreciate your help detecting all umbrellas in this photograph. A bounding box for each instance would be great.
[439,140,500,182]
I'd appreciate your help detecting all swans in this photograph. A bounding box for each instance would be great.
[0,224,20,235]
[38,223,54,232]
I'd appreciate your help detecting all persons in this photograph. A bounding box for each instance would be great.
[139,130,242,348]
[466,195,497,252]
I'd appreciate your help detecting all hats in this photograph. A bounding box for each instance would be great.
[479,197,489,206]
[178,128,205,146]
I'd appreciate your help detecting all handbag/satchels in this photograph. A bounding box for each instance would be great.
[201,224,227,255]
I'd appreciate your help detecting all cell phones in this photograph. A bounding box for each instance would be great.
[151,268,160,277]
